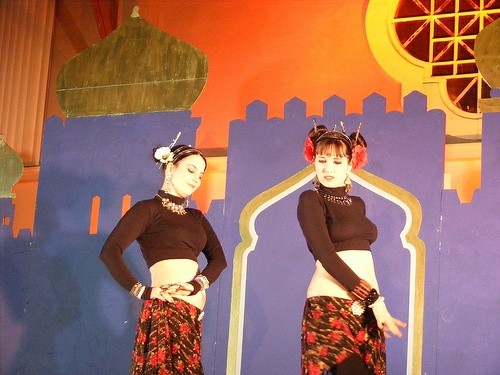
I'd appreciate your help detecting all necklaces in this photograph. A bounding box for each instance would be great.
[313,186,353,206]
[154,191,189,216]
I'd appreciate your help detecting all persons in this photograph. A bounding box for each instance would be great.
[99,132,228,375]
[296,118,408,375]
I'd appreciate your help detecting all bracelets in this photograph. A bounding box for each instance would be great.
[194,274,209,292]
[129,282,145,299]
[351,279,384,308]
